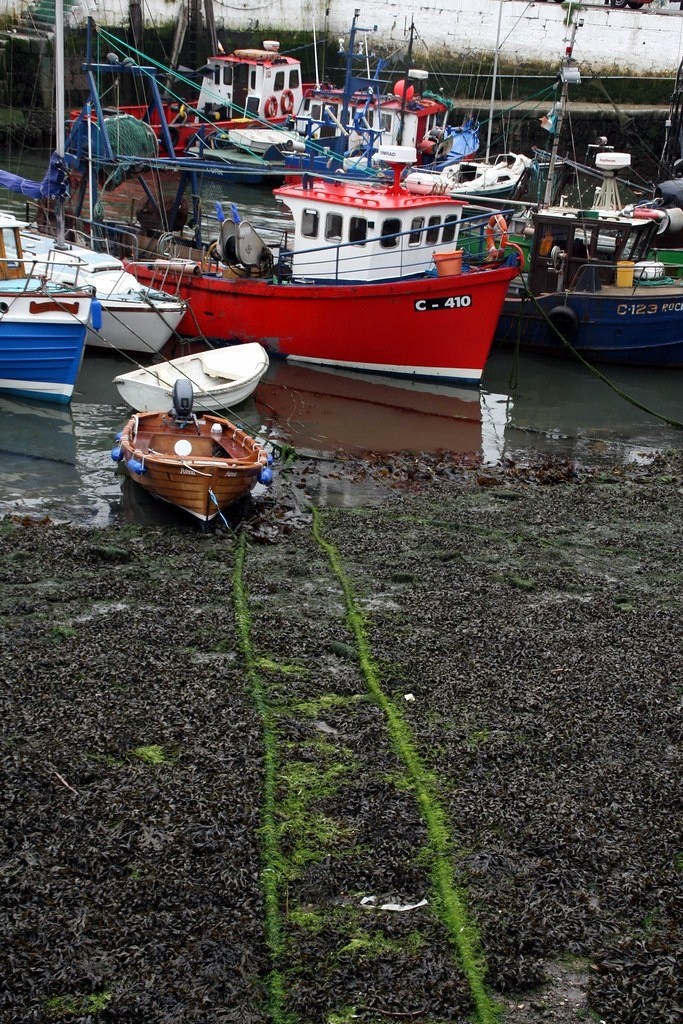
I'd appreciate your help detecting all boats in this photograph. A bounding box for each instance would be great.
[69,38,338,159]
[114,174,528,389]
[112,340,270,414]
[119,411,269,522]
[179,15,482,188]
[1,218,100,408]
[459,19,683,358]
[401,147,535,205]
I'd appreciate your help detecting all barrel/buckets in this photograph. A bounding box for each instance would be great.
[432,250,463,277]
[617,261,634,287]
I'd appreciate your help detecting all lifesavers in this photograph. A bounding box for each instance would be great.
[264,95,279,118]
[280,89,295,114]
[623,207,666,219]
[486,214,508,260]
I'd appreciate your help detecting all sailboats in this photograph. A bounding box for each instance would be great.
[1,0,187,355]
